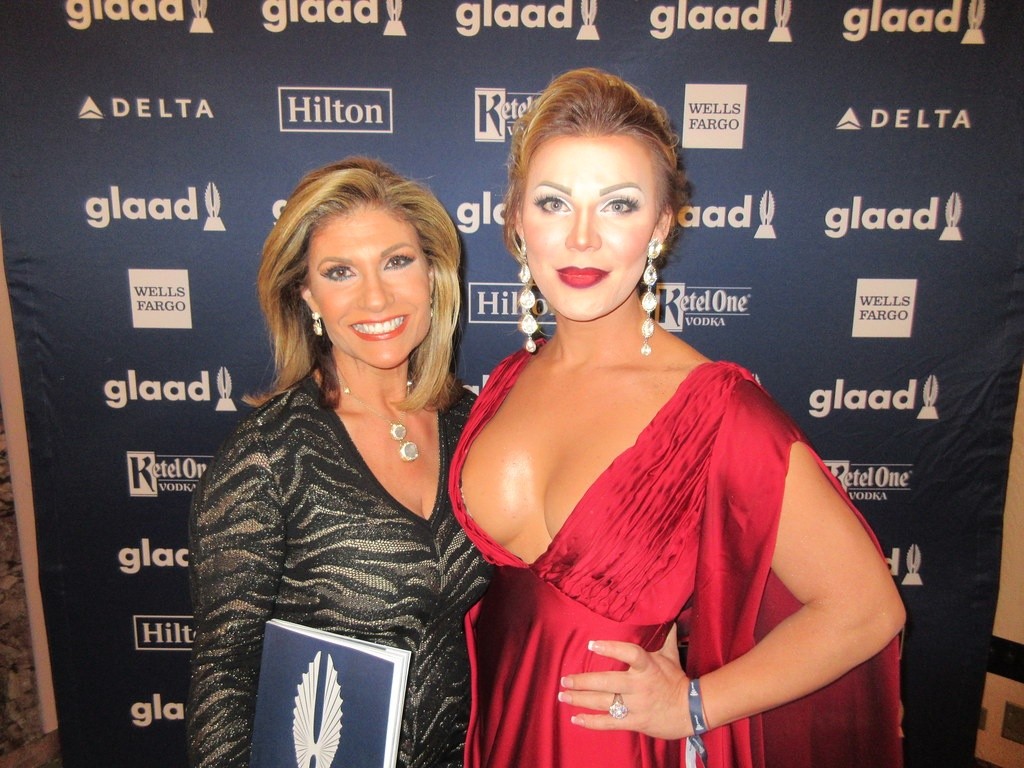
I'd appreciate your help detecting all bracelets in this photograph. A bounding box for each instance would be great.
[689,678,708,768]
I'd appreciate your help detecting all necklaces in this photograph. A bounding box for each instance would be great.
[341,377,420,461]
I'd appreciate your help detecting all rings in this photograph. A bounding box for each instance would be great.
[609,693,627,720]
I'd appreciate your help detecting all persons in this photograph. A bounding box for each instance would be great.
[191,155,495,768]
[450,70,905,768]
